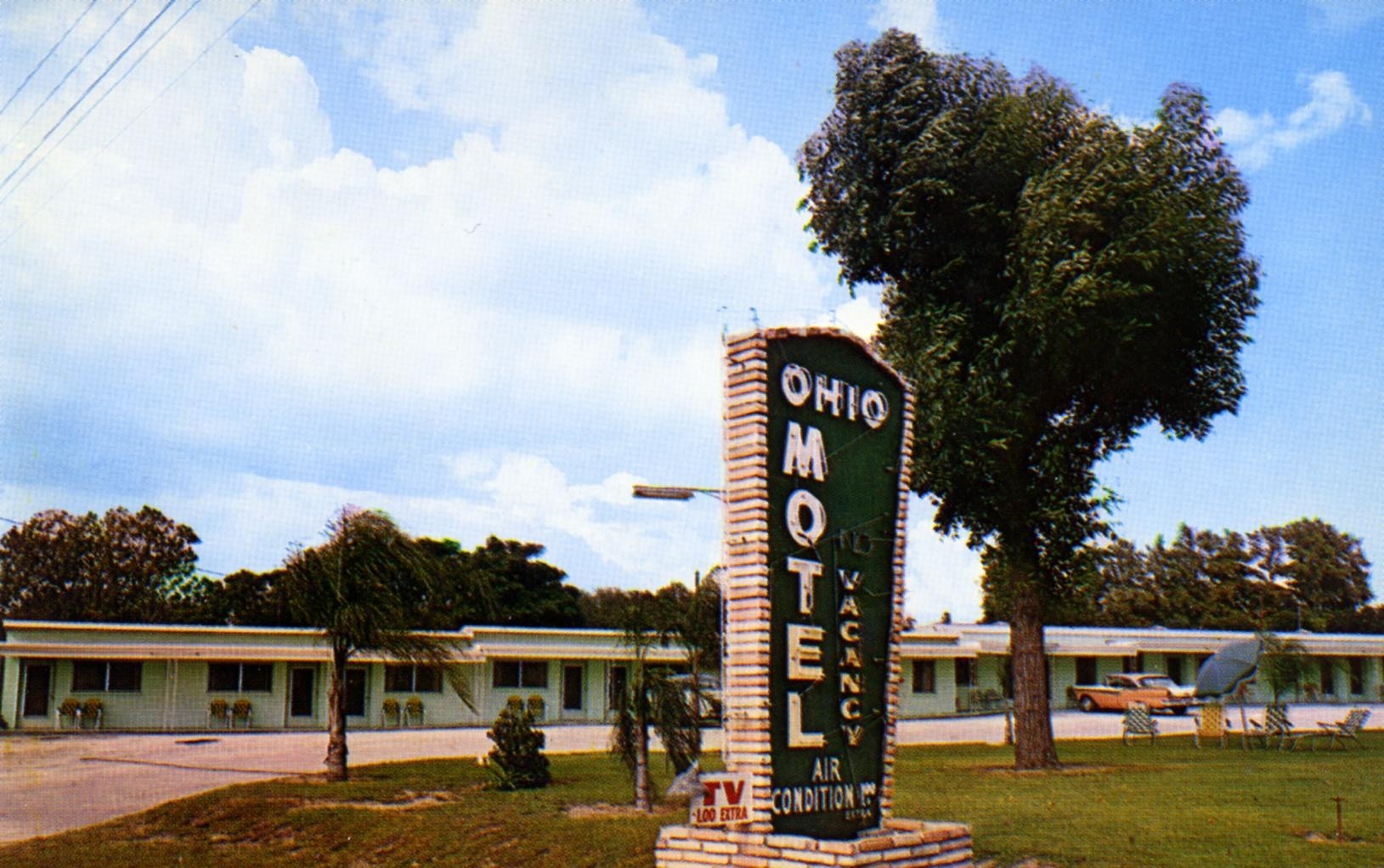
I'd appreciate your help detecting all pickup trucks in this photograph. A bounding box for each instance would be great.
[1071,670,1201,715]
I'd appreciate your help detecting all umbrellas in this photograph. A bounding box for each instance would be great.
[1194,637,1267,750]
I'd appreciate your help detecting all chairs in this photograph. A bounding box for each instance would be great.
[1121,701,1157,746]
[528,693,546,722]
[402,696,424,726]
[382,699,402,729]
[205,698,229,728]
[1313,708,1369,751]
[507,695,523,714]
[54,698,79,728]
[1192,703,1231,749]
[1251,701,1292,749]
[229,699,254,728]
[78,698,104,729]
